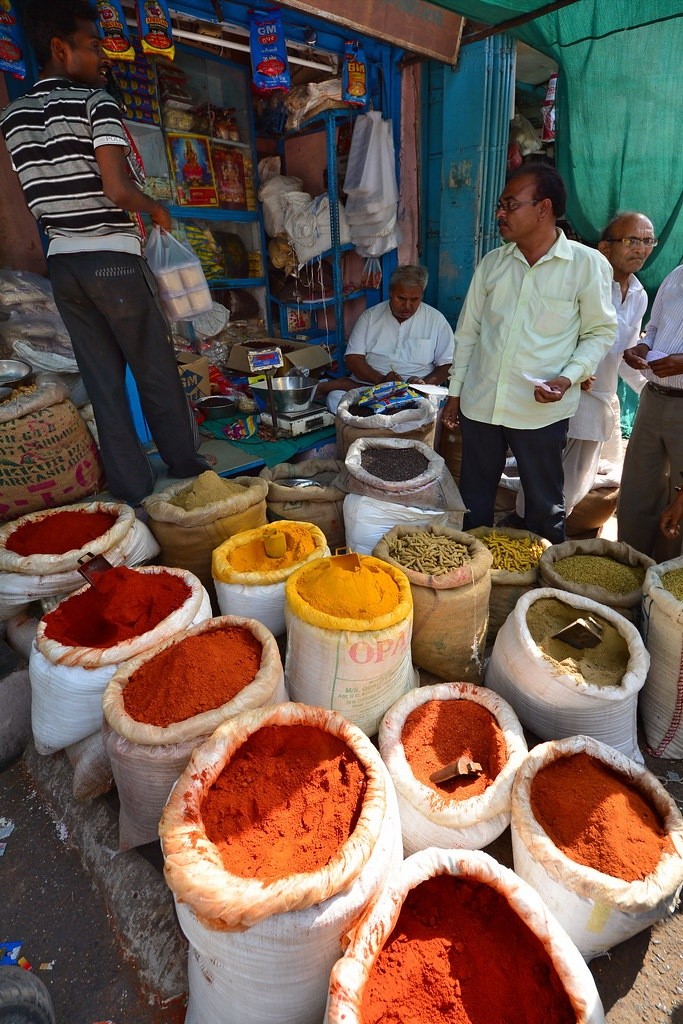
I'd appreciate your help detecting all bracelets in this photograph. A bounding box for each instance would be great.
[674,486,683,492]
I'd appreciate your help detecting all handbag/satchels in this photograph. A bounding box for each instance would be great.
[144,224,215,321]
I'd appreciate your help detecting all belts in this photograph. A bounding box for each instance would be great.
[646,380,683,398]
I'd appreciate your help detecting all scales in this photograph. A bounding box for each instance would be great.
[246,344,336,440]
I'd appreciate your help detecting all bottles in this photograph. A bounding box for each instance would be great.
[156,260,211,320]
[217,126,238,141]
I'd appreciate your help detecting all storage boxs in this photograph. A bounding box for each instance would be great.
[227,334,334,377]
[174,351,212,403]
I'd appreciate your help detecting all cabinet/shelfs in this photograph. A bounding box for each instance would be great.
[96,20,398,479]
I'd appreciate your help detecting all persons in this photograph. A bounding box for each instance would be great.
[442,162,618,545]
[617,264,683,566]
[0,0,217,507]
[344,265,455,386]
[561,212,658,518]
[659,486,683,539]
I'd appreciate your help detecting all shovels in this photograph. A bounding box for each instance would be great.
[284,479,321,488]
[329,546,361,572]
[263,528,287,558]
[77,552,114,589]
[551,617,604,649]
[430,757,483,784]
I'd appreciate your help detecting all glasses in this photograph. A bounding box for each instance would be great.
[606,237,659,247]
[494,200,538,211]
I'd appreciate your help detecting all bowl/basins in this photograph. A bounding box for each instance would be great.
[196,395,238,418]
[0,359,32,385]
[248,377,319,412]
